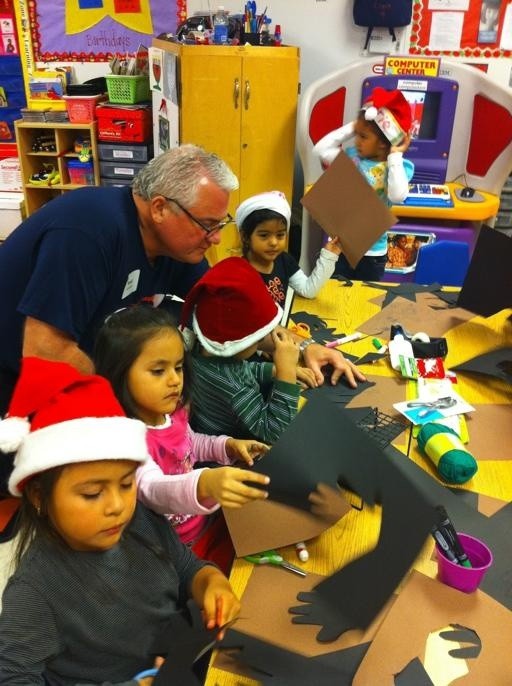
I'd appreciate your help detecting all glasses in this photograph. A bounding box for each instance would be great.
[164,196,233,232]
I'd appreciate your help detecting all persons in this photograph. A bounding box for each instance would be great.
[480,0,499,43]
[95,308,270,574]
[178,256,318,447]
[6,37,14,53]
[0,125,10,142]
[0,95,6,106]
[407,237,427,256]
[312,86,414,282]
[1,143,366,417]
[234,189,344,309]
[387,234,410,270]
[3,20,10,32]
[0,358,245,681]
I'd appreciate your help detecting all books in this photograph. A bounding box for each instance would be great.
[29,67,70,99]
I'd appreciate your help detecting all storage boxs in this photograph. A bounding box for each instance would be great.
[98,146,147,163]
[0,158,23,193]
[103,181,132,189]
[100,161,144,179]
[0,190,24,209]
[95,103,152,143]
[62,95,110,123]
[68,161,93,184]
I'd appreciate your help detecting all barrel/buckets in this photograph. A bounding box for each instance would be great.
[435,532,493,594]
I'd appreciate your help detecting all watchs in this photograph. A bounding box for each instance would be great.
[295,339,314,355]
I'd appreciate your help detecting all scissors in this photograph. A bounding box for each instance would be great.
[245,550,308,578]
[133,638,215,685]
[290,323,312,339]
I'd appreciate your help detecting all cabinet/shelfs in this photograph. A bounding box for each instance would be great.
[15,119,97,220]
[150,38,299,273]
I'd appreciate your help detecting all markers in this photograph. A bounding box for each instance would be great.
[430,527,460,567]
[240,2,258,34]
[325,332,360,347]
[372,344,388,365]
[295,540,309,563]
[372,339,382,350]
[435,506,474,568]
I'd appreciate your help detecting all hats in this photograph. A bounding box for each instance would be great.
[235,190,292,234]
[360,87,412,146]
[0,357,147,498]
[177,256,284,357]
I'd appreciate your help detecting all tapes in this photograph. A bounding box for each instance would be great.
[409,331,430,345]
